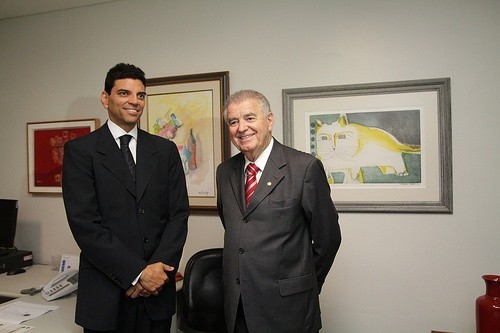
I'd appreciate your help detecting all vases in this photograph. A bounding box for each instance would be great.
[476,275,500,333]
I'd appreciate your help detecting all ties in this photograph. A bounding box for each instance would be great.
[244,163,260,209]
[119,134,136,182]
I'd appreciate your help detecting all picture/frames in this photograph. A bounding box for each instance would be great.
[26,118,100,194]
[281,77,453,214]
[137,71,231,216]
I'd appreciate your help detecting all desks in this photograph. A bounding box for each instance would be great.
[0,264,186,333]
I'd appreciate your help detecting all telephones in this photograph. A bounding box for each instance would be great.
[41,268,79,302]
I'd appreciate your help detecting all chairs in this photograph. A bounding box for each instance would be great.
[177,248,228,333]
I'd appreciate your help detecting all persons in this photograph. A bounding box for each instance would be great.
[62,63,190,333]
[217,90,341,333]
